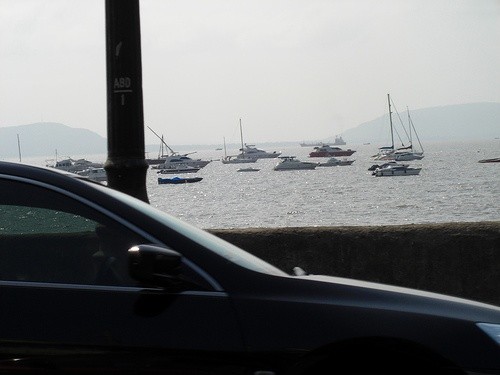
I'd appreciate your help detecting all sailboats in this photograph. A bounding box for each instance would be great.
[145,124,213,175]
[220,118,260,165]
[368,94,426,178]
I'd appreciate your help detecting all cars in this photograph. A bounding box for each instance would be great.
[2,159,499,374]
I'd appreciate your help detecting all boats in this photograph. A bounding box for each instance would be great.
[308,143,357,157]
[317,158,356,167]
[272,157,318,172]
[237,143,283,159]
[44,148,108,181]
[158,177,203,184]
